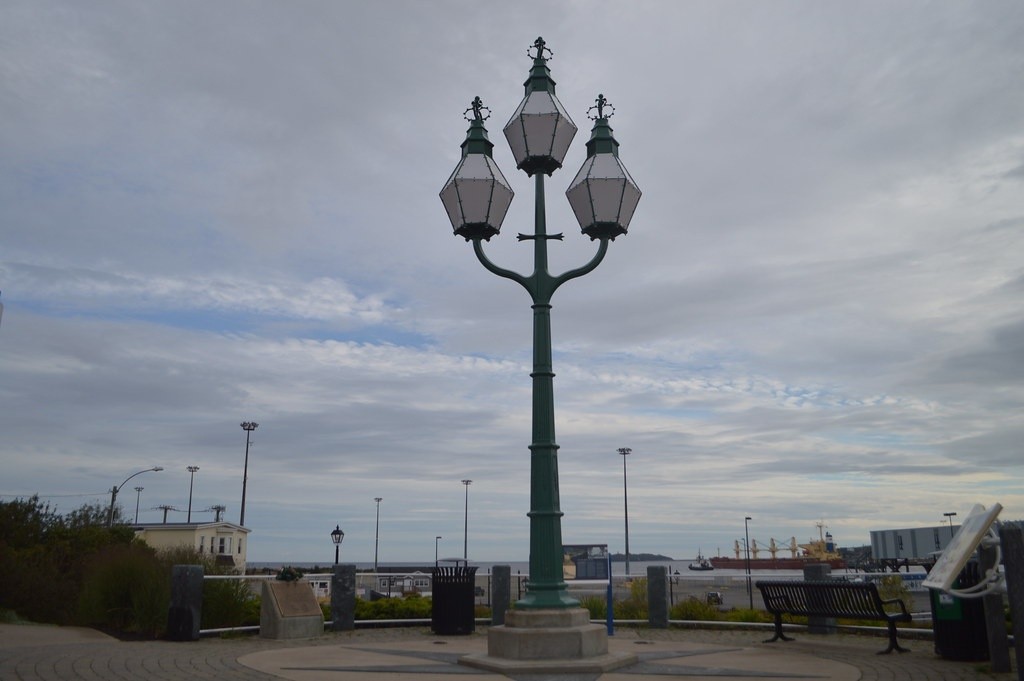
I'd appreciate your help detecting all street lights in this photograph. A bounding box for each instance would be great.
[108,467,164,527]
[239,421,258,526]
[435,536,442,567]
[944,512,957,538]
[375,498,382,571]
[461,480,472,566]
[617,447,632,574]
[745,517,753,610]
[187,466,200,522]
[135,486,144,524]
[439,35,643,609]
[331,525,344,564]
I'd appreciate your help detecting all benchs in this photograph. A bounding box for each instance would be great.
[755,579,913,657]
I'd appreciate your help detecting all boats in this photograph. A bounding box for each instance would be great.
[689,559,714,570]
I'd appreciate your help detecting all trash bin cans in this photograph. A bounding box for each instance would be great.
[918,559,991,663]
[427,565,480,635]
